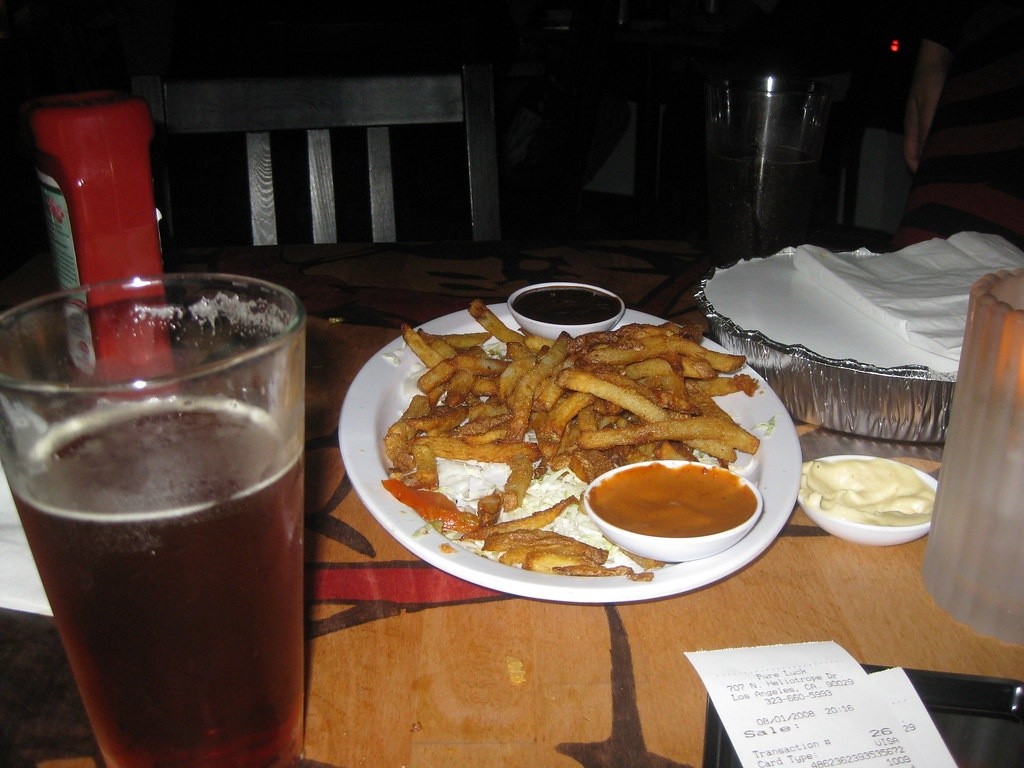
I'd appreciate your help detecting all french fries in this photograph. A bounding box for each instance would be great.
[384,298,762,581]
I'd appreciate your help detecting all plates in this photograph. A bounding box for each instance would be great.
[338,303,804,606]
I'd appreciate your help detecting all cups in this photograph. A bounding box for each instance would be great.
[916,266,1024,648]
[706,76,833,266]
[0,272,307,768]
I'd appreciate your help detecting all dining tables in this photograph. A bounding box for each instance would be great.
[0,239,1024,768]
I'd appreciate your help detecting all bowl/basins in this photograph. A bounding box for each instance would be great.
[582,459,765,565]
[507,282,626,340]
[799,452,941,548]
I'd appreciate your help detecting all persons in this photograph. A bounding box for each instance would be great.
[890,1,1024,254]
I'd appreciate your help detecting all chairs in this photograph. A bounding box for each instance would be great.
[134,63,501,245]
[267,15,477,78]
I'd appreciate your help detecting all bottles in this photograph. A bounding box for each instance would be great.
[30,90,174,377]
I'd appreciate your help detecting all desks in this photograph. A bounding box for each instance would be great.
[526,28,721,201]
